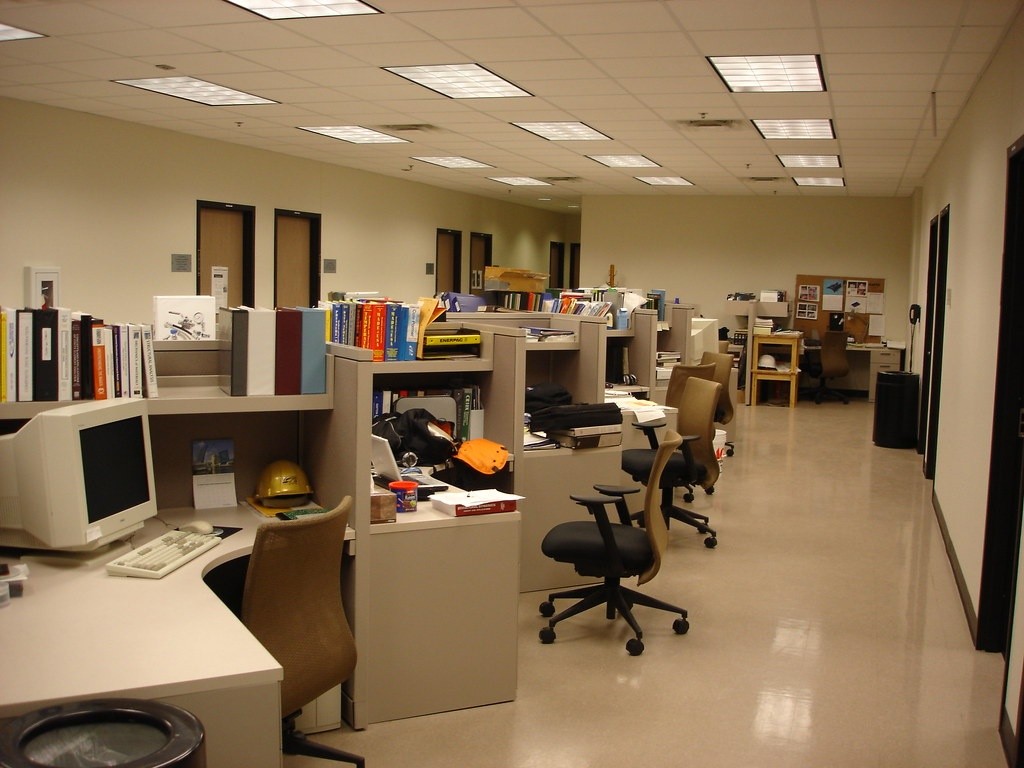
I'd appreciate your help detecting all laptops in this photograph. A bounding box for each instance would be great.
[371,434,449,491]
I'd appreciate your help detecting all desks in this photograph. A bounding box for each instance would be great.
[0,305,695,768]
[751,334,802,408]
[805,345,901,404]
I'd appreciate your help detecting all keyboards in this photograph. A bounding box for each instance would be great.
[106,530,222,579]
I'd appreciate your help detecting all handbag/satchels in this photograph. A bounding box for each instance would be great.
[371,408,455,467]
[528,403,623,432]
[524,382,572,413]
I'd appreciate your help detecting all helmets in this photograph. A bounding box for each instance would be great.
[255,460,314,498]
[757,355,776,368]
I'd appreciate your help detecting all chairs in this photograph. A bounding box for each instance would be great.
[807,331,850,405]
[666,361,716,440]
[622,377,723,550]
[241,494,365,768]
[701,352,737,457]
[538,426,688,657]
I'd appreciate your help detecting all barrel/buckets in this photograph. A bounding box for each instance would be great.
[713,429,727,472]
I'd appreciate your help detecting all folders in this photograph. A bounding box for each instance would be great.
[216,305,327,396]
[433,291,487,312]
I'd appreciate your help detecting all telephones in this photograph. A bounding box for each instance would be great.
[909,304,921,325]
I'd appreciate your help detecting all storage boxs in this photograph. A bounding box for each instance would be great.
[484,266,551,293]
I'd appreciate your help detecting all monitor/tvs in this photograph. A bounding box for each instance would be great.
[0,398,158,566]
[691,318,719,366]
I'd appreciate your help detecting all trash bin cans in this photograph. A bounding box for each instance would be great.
[712,429,727,478]
[0,698,207,768]
[872,369,920,450]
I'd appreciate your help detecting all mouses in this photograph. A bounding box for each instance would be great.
[178,520,213,534]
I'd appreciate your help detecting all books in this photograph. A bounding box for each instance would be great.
[623,347,630,375]
[0,306,158,404]
[454,388,472,441]
[643,289,666,321]
[551,424,623,450]
[754,317,774,328]
[656,351,682,368]
[505,291,613,318]
[432,495,516,517]
[320,300,420,362]
